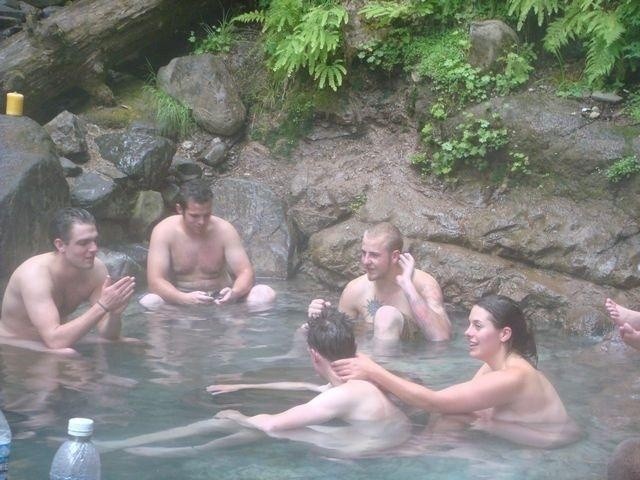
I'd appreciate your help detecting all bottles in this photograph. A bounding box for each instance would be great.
[51,417,103,479]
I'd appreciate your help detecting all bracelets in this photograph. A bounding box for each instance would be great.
[97,300,110,312]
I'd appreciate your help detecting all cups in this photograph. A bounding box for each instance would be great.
[6,92,24,116]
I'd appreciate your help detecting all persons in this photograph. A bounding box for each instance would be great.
[606,436,640,480]
[0,207,145,390]
[93,306,413,460]
[331,294,579,458]
[139,180,277,361]
[605,298,640,350]
[271,222,452,363]
